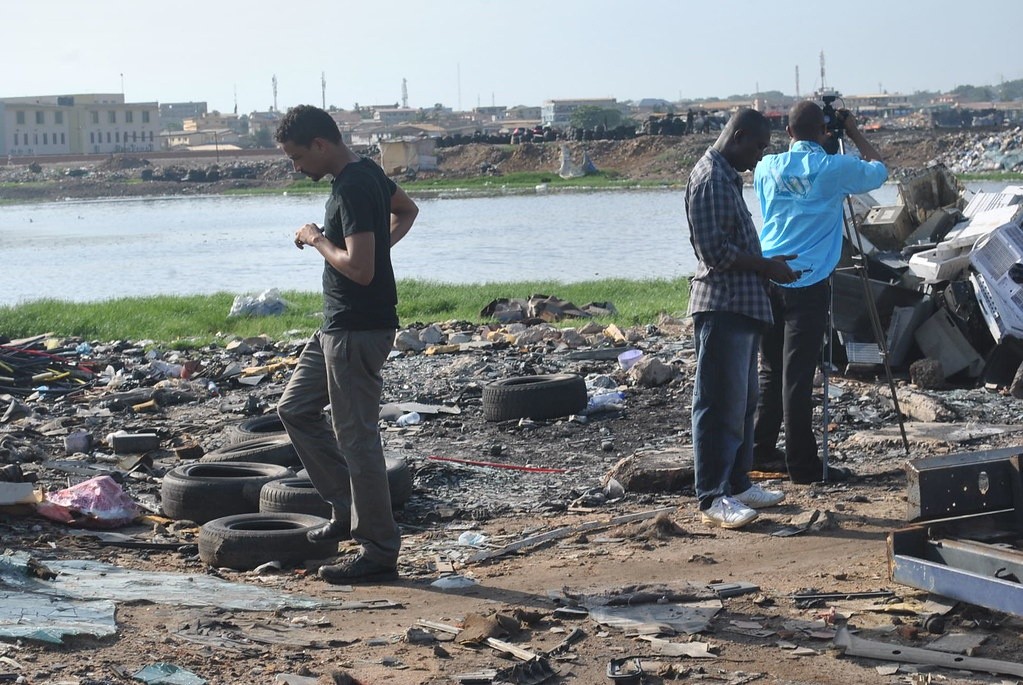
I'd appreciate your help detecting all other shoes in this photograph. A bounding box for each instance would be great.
[791,465,850,484]
[751,450,787,472]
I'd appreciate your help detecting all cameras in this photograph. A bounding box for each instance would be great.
[822,95,847,138]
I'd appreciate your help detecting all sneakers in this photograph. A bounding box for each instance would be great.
[733,483,785,508]
[702,492,760,528]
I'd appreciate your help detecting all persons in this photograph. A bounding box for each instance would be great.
[685,108,694,134]
[752,100,887,485]
[274,103,420,585]
[684,108,785,527]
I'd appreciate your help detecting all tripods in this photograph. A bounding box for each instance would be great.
[820,128,910,484]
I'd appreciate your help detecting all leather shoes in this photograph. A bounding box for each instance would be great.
[318,551,399,585]
[306,518,353,543]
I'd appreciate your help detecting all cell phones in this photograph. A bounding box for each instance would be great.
[298,224,325,244]
